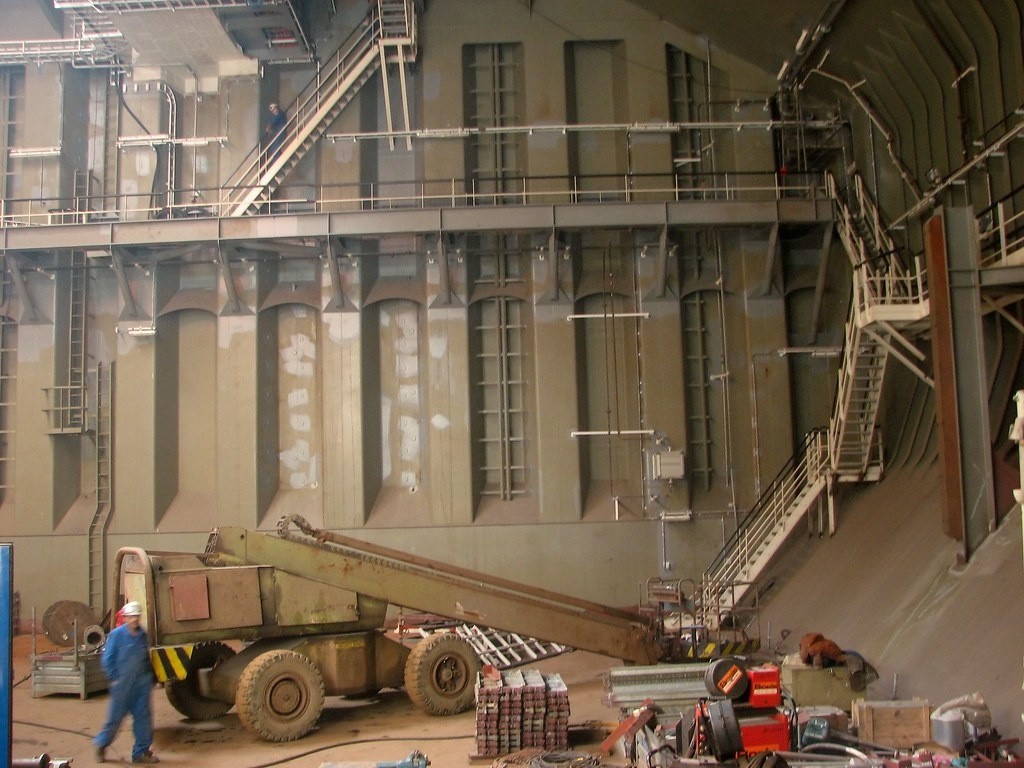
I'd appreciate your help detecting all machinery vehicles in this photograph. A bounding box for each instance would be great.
[107,515,764,745]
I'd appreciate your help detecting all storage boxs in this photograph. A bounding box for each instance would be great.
[780,665,867,711]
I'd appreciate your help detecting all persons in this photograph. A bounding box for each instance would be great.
[90,601,160,764]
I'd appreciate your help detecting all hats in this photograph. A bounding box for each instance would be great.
[120,601,143,615]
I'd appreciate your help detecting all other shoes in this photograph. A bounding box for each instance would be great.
[132,749,161,763]
[93,746,106,763]
